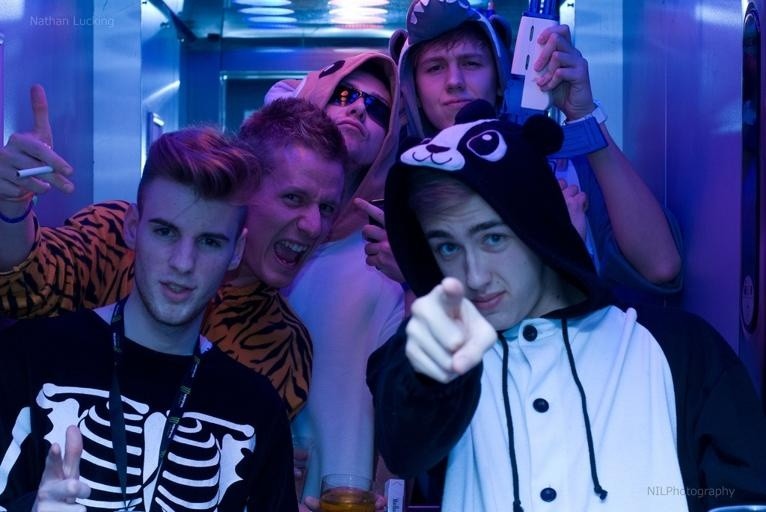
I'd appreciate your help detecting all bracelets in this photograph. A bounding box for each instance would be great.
[562,99,609,125]
[0,193,38,223]
[401,281,410,292]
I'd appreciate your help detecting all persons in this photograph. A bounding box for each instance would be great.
[259,49,416,512]
[15,164,55,178]
[0,81,350,503]
[0,120,302,512]
[364,99,765,512]
[387,0,687,295]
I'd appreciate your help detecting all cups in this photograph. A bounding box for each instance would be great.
[319,473,378,512]
[291,433,316,509]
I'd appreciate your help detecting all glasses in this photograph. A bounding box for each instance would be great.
[327,81,391,134]
